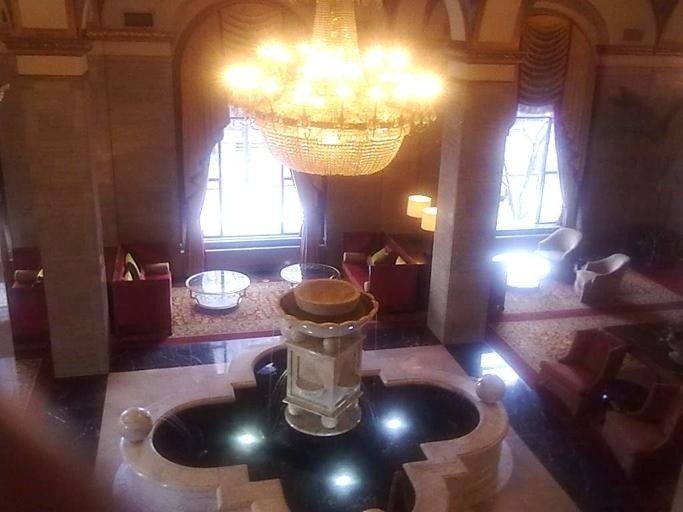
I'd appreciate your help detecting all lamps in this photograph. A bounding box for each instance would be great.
[407,195,432,241]
[421,206,437,234]
[216,0,450,176]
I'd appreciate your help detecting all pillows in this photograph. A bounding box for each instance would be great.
[394,256,407,265]
[342,251,366,263]
[125,271,133,281]
[369,248,388,266]
[146,262,169,273]
[126,252,142,280]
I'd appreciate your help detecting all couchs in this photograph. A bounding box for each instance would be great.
[389,229,431,311]
[341,229,421,311]
[111,243,172,336]
[4,262,48,351]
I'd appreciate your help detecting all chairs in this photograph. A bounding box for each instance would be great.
[574,253,630,304]
[597,379,682,485]
[536,227,582,280]
[537,326,628,420]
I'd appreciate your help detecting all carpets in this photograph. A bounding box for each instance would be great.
[115,276,292,346]
[0,351,51,436]
[485,267,683,390]
[604,317,682,378]
